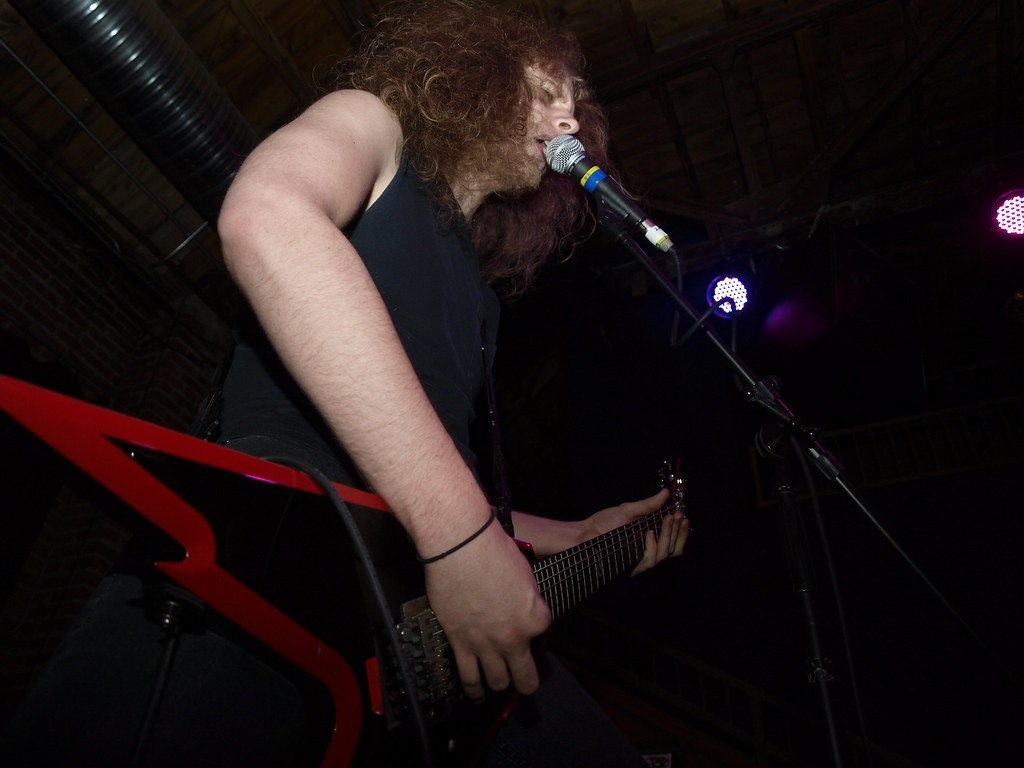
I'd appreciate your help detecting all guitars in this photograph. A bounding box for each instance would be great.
[0,372,695,768]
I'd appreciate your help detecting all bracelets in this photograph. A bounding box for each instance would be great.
[416,508,496,563]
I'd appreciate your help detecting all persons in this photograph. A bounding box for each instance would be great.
[218,2,689,768]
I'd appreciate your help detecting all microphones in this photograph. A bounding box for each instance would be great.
[546,135,678,257]
